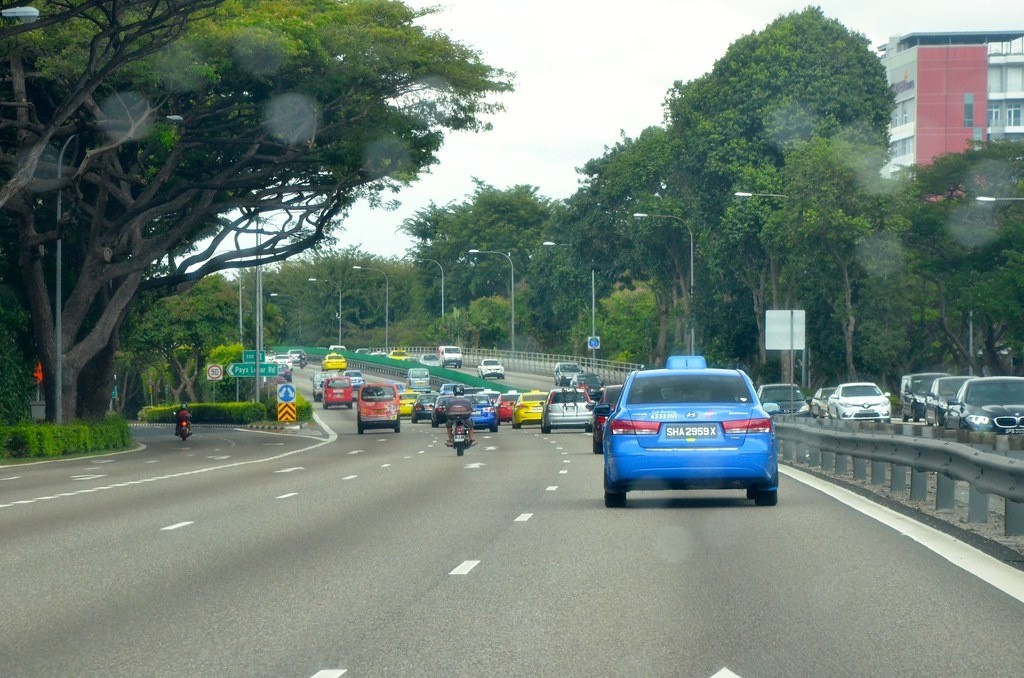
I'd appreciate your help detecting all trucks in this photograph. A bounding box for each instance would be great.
[436,346,463,369]
[310,371,339,402]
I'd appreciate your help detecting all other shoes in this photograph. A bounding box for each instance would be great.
[445,440,452,447]
[472,440,479,446]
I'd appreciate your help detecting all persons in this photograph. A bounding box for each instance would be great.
[298,351,305,361]
[175,401,192,436]
[445,383,477,446]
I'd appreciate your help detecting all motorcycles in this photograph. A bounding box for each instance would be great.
[173,410,194,441]
[441,399,479,456]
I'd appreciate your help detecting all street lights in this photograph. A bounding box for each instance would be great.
[633,213,695,357]
[469,249,515,351]
[309,278,341,345]
[353,266,388,353]
[54,115,184,424]
[404,257,444,319]
[271,293,302,335]
[542,242,595,368]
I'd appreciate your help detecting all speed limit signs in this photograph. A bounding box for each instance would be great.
[207,364,223,379]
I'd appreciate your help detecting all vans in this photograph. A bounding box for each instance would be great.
[900,373,951,424]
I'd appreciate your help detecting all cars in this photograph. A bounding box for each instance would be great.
[756,382,892,423]
[925,375,1024,435]
[585,356,782,509]
[268,345,604,435]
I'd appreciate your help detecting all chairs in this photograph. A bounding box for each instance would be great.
[636,384,664,403]
[710,385,736,403]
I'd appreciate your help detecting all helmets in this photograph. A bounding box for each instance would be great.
[453,384,466,395]
[180,403,187,409]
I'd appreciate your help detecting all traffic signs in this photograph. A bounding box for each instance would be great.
[226,363,278,377]
